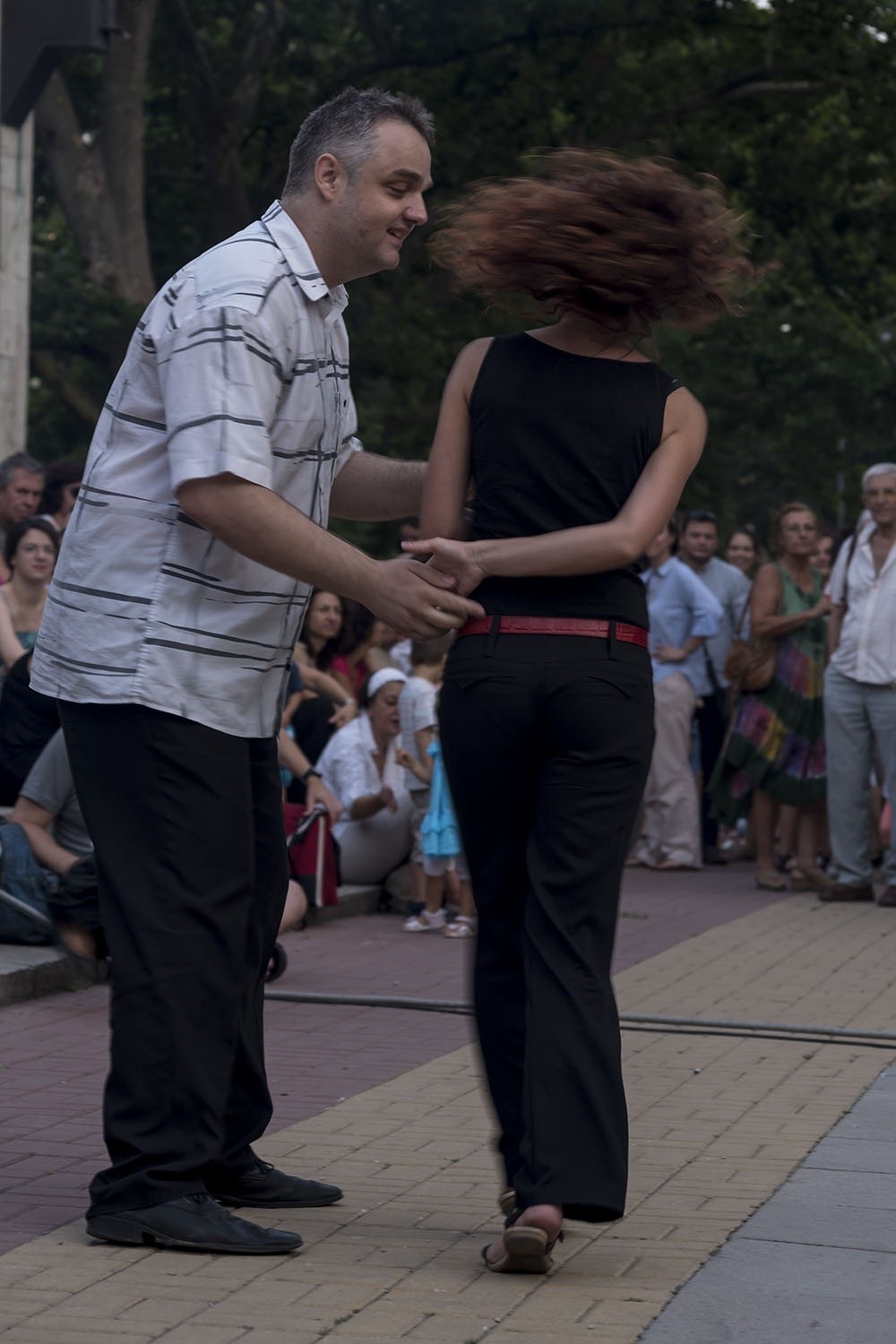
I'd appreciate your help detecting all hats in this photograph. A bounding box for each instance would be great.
[368,667,407,697]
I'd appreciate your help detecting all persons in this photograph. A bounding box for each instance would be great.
[0,450,484,982]
[399,151,764,1277]
[29,84,482,1256]
[628,461,896,911]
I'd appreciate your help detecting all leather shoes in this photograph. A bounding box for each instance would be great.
[84,1192,304,1255]
[212,1158,344,1209]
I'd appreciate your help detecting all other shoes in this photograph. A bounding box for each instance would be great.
[818,884,876,902]
[789,867,834,892]
[878,885,896,907]
[753,860,788,892]
[265,943,287,983]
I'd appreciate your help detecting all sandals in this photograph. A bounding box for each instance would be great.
[401,908,447,932]
[444,916,476,939]
[498,1188,518,1216]
[480,1201,566,1274]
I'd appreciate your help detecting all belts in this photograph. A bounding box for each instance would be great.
[454,614,648,650]
